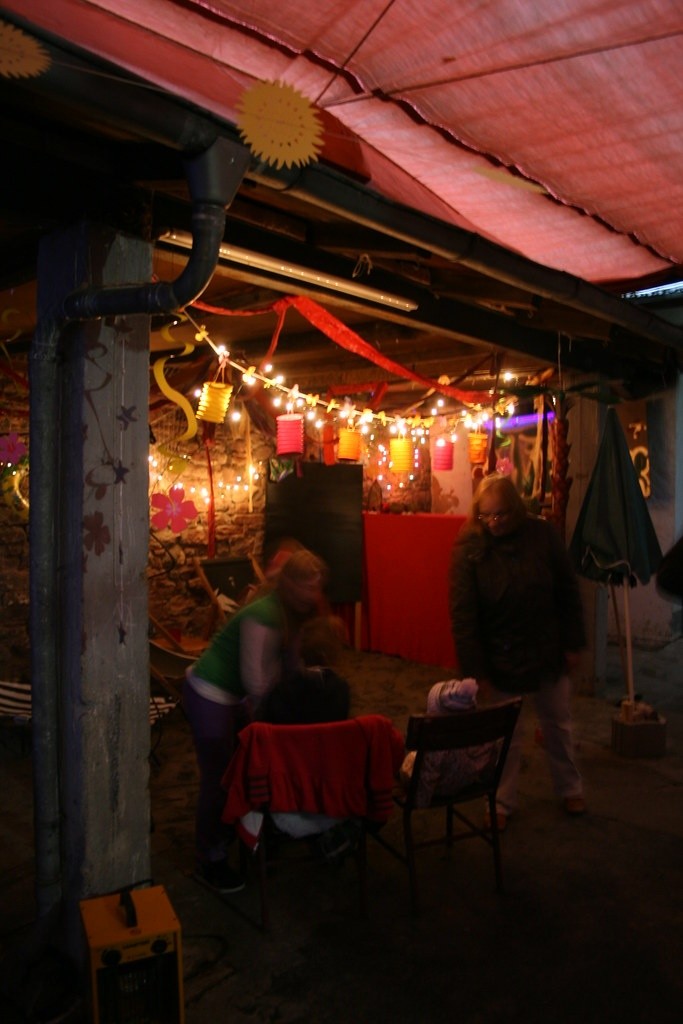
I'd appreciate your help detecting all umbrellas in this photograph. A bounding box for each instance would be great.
[564,380,668,758]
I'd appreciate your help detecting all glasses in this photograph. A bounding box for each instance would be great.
[476,513,510,521]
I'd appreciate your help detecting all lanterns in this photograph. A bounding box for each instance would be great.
[433,440,454,472]
[467,431,488,472]
[196,381,234,426]
[276,413,304,457]
[389,435,415,474]
[337,428,362,460]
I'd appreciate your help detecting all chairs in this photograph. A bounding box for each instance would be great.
[365,692,528,905]
[189,714,399,964]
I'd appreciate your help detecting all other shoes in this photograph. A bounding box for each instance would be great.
[565,793,583,815]
[484,807,508,829]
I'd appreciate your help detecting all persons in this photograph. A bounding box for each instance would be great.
[257,613,356,726]
[448,471,590,836]
[398,677,482,784]
[180,540,325,894]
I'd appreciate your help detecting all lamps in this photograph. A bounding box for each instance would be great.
[172,316,523,492]
